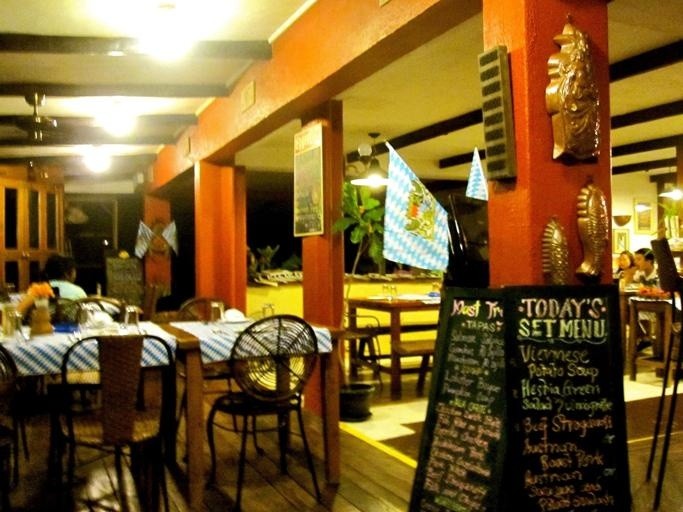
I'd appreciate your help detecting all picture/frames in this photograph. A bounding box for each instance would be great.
[634,196,656,234]
[612,228,630,254]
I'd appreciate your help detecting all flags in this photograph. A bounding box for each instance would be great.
[382,140,488,274]
[134,220,179,259]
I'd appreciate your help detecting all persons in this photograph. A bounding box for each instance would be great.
[616,248,660,351]
[18,253,88,327]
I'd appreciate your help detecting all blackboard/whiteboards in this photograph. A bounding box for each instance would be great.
[408,287,504,512]
[503,284,632,512]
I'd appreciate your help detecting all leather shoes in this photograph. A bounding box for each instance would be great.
[637,339,653,351]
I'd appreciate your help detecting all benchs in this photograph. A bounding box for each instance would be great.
[393,340,440,398]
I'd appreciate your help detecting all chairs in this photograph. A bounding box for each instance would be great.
[37,295,132,477]
[174,298,250,435]
[207,313,321,512]
[48,336,177,510]
[341,309,384,393]
[0,345,32,509]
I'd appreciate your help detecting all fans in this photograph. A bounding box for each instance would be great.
[0,91,107,140]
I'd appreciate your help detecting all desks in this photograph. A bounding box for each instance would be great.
[1,317,175,509]
[159,318,342,509]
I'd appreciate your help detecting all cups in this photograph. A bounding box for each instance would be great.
[262,303,274,319]
[381,284,400,304]
[210,301,225,327]
[123,305,140,336]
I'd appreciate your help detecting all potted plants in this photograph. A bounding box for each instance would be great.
[329,179,386,421]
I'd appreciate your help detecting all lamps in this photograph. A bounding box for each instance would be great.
[350,132,391,186]
